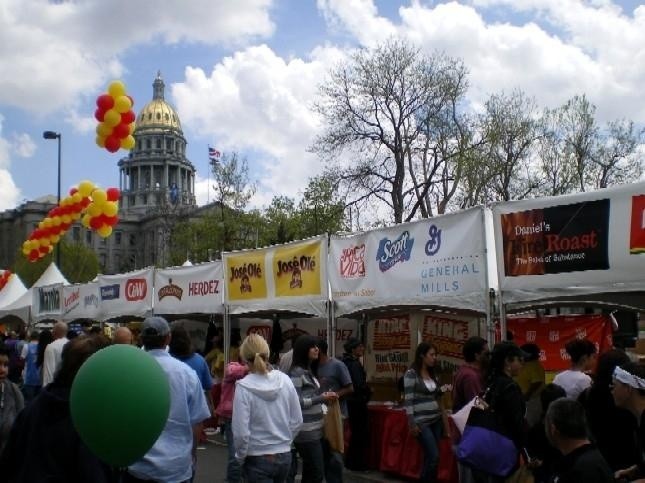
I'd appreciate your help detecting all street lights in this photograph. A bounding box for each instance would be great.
[43,131,62,269]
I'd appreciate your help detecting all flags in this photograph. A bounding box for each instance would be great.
[208,147,221,159]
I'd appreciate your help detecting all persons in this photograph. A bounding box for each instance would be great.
[448,334,645,483]
[403,339,455,482]
[1,314,222,481]
[215,333,377,482]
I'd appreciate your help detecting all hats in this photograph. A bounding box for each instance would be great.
[140,316,170,337]
[343,337,363,352]
[492,341,531,356]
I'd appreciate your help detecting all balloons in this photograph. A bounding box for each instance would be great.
[21,179,120,261]
[93,80,137,153]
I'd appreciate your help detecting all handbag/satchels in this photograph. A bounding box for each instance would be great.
[451,395,494,436]
[457,405,517,475]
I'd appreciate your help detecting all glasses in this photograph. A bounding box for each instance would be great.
[609,382,617,390]
